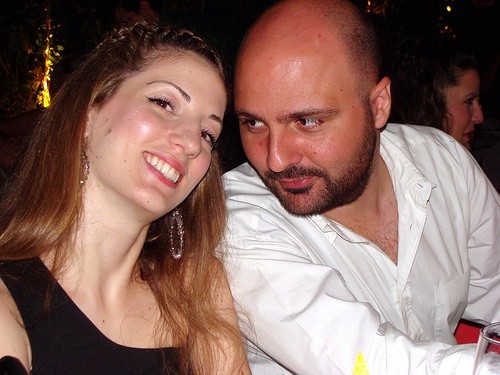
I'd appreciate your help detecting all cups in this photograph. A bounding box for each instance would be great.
[473,322,500,373]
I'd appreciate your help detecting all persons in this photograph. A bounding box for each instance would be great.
[209,0,500,375]
[388,37,484,152]
[0,18,258,375]
